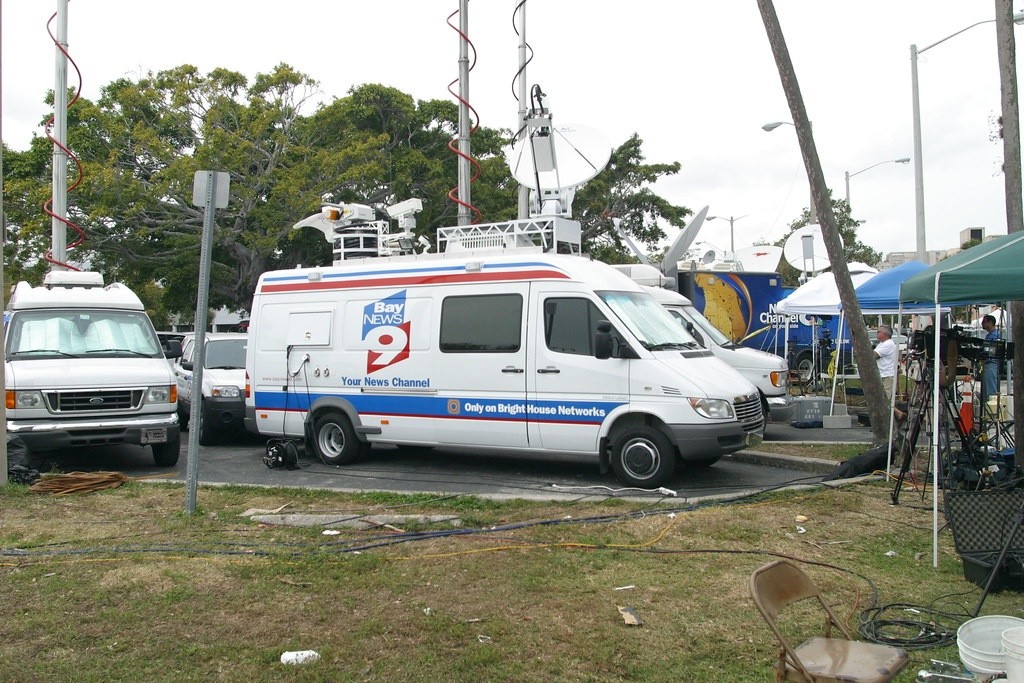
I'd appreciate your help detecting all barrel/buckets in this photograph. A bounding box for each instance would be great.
[957,615,1024,683]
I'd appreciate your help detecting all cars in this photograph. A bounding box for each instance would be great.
[867,328,910,356]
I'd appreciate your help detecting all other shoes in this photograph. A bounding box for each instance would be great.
[897,413,907,428]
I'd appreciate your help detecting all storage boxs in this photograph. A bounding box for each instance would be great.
[989,395,1007,419]
[946,488,1024,595]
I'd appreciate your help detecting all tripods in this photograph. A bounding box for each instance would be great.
[779,341,831,397]
[889,357,1013,501]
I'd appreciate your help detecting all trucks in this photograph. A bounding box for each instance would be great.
[674,271,855,385]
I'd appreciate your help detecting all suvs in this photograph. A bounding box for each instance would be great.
[4,280,183,469]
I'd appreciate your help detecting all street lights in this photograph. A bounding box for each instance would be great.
[844,158,911,220]
[909,11,1024,265]
[704,212,749,253]
[760,119,821,223]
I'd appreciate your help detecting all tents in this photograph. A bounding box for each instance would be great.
[774,262,954,394]
[828,260,1012,417]
[887,230,1024,567]
[971,307,1008,331]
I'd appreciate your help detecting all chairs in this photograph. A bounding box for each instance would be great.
[750,559,909,683]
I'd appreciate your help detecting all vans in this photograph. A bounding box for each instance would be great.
[155,330,248,446]
[244,247,795,488]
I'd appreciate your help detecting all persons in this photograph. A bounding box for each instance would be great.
[872,323,899,404]
[979,313,1001,404]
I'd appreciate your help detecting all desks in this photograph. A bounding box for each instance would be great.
[821,373,860,405]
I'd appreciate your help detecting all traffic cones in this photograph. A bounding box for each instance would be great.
[956,376,976,439]
